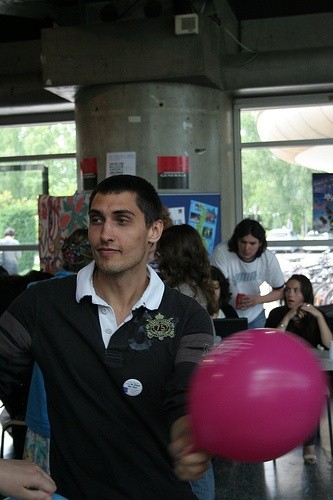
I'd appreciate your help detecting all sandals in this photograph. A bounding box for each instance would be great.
[303,445,317,464]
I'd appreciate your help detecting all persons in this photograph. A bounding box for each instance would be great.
[1,224,215,490]
[27,176,239,499]
[210,218,285,327]
[1,174,214,500]
[1,458,57,500]
[264,274,333,464]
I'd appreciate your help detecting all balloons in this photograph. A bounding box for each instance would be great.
[187,327,328,464]
